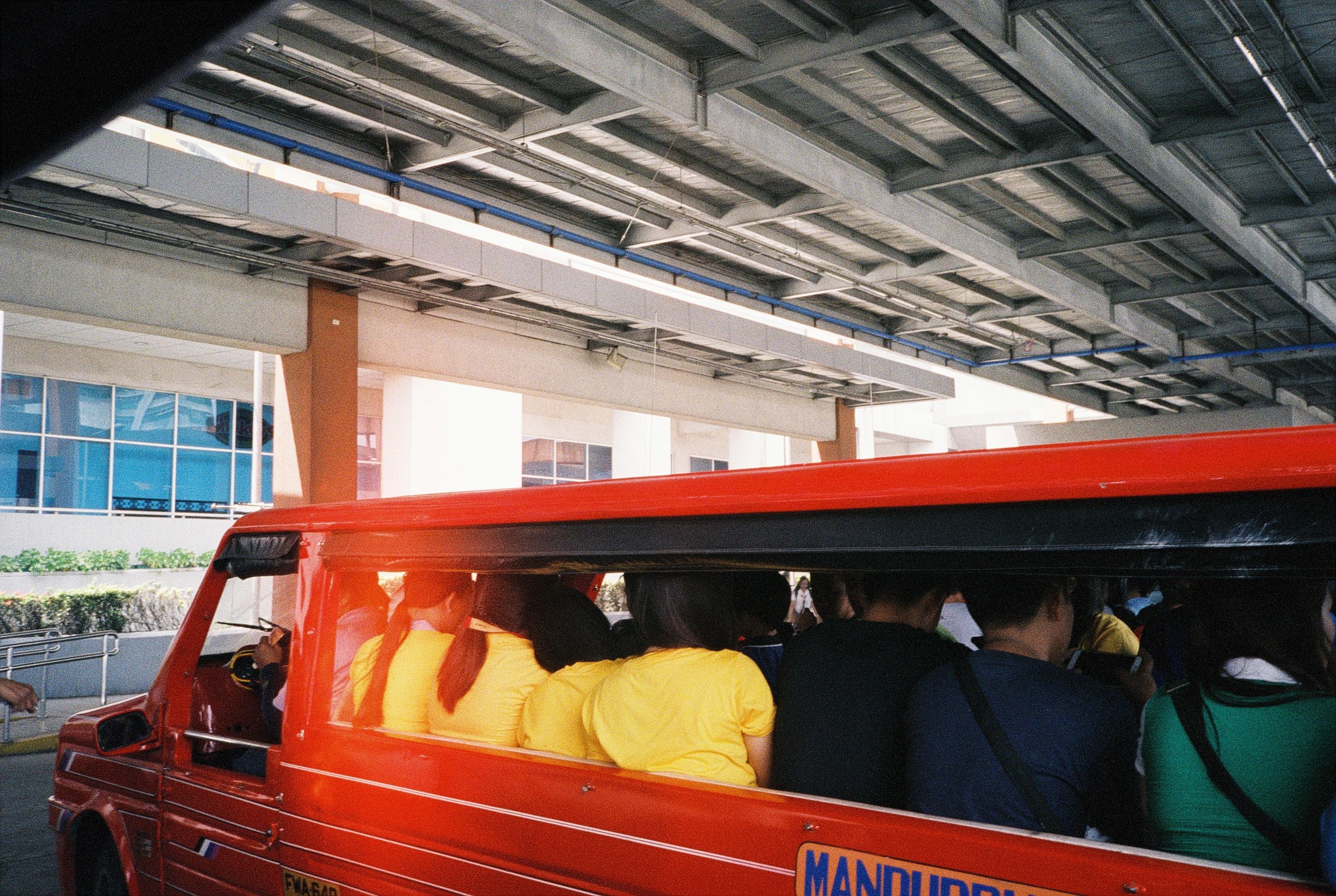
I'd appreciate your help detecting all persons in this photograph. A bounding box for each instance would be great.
[1135,571,1336,885]
[1065,569,1199,707]
[608,617,653,660]
[905,572,1151,851]
[582,568,776,788]
[735,569,794,695]
[776,571,973,809]
[786,576,818,633]
[347,570,474,736]
[434,572,561,747]
[230,570,389,792]
[0,678,40,713]
[514,583,624,760]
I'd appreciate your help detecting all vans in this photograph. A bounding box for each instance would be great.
[48,425,1336,895]
